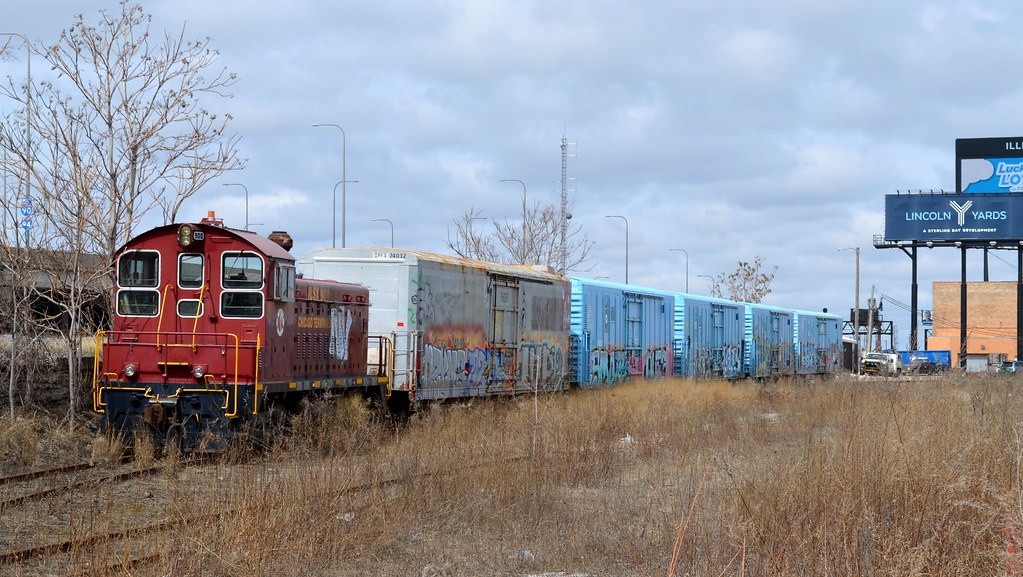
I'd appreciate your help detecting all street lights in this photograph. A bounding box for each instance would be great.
[332,179,359,249]
[0,32,33,247]
[311,122,347,248]
[498,178,527,265]
[604,215,629,285]
[668,248,689,295]
[696,274,715,298]
[373,218,395,248]
[222,182,250,231]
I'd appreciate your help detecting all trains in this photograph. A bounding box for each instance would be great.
[91,211,846,464]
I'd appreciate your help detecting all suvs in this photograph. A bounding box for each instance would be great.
[861,352,896,376]
[996,360,1023,375]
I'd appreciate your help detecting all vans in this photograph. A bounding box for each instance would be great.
[887,353,902,374]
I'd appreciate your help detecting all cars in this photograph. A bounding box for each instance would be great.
[909,358,937,375]
